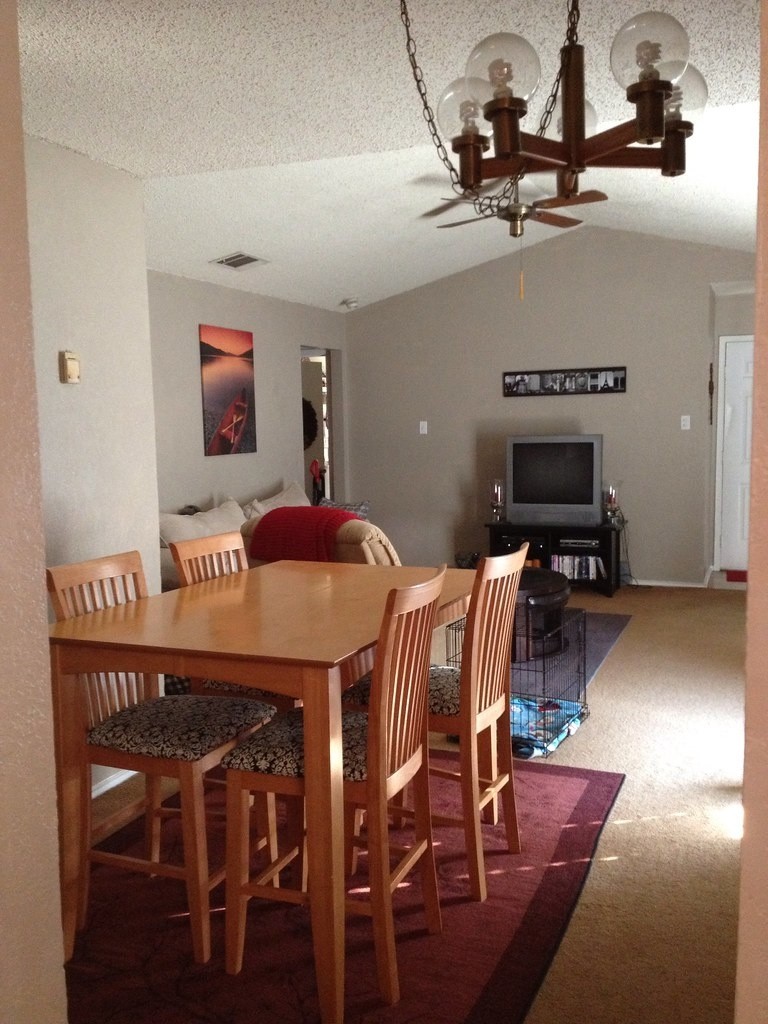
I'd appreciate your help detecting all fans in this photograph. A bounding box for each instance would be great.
[437,175,608,237]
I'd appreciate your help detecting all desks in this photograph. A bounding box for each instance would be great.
[45,559,479,1024]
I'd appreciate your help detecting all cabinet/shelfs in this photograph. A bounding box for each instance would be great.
[487,520,621,598]
[502,367,627,397]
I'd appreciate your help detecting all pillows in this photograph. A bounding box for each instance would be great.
[160,495,248,551]
[243,482,311,521]
[317,497,371,521]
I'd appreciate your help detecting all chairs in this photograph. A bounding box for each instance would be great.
[46,551,279,962]
[167,530,304,717]
[220,565,446,1004]
[345,541,531,904]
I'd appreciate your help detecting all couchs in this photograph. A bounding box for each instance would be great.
[159,481,403,595]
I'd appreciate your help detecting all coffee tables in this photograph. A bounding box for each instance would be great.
[510,568,572,664]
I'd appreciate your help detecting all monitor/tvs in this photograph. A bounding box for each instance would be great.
[505,434,603,527]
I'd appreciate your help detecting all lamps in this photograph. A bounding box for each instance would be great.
[485,478,505,523]
[602,480,626,528]
[394,0,709,216]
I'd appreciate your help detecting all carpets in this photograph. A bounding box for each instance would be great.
[510,601,632,702]
[61,750,627,1024]
